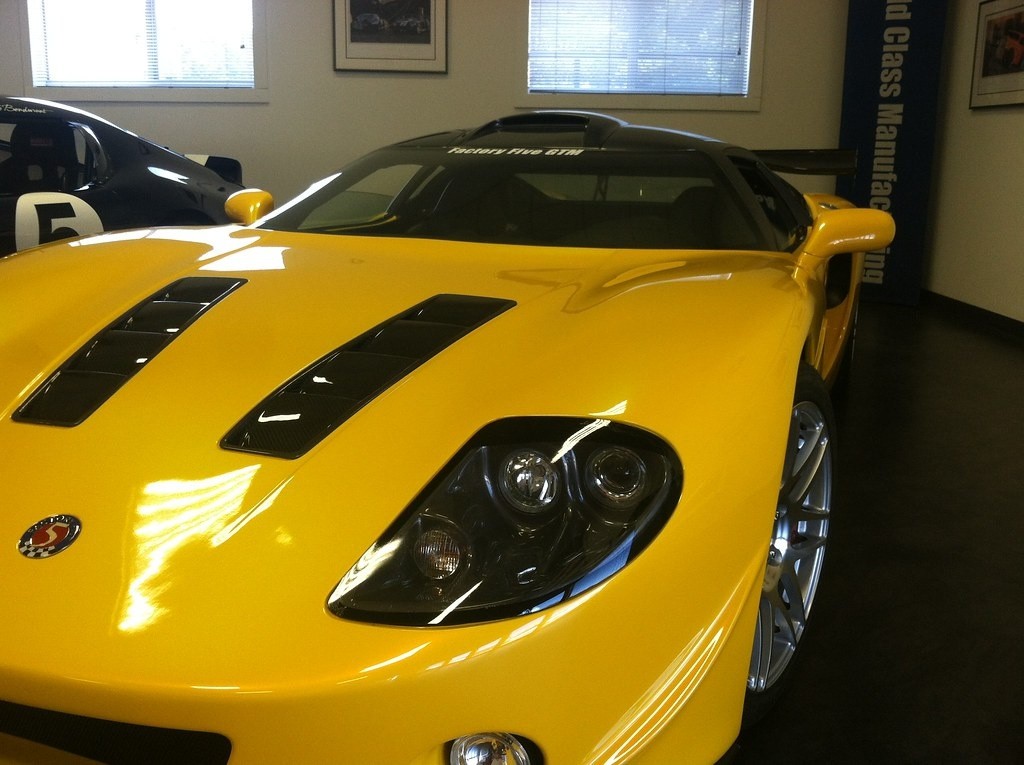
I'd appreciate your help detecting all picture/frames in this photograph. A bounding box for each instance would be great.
[967,0,1024,110]
[330,0,449,75]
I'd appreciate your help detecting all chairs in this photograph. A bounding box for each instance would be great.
[408,171,534,243]
[0,122,80,194]
[668,185,755,248]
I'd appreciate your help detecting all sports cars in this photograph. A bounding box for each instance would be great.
[0,96,248,257]
[0,110,896,765]
